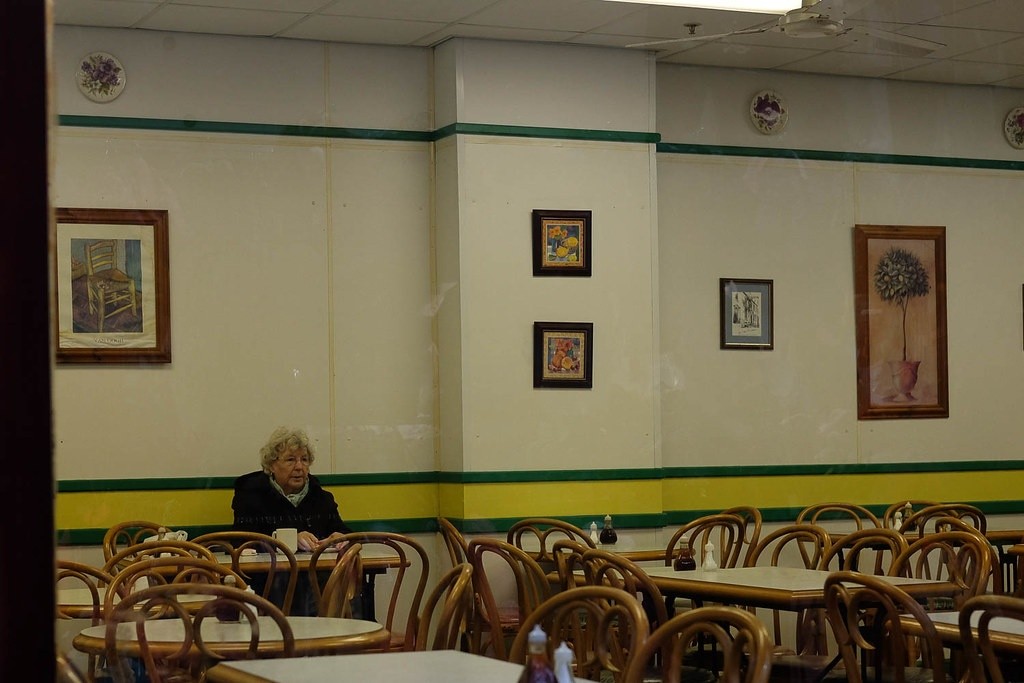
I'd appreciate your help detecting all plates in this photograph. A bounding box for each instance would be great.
[749,90,788,134]
[75,52,127,103]
[1004,107,1024,150]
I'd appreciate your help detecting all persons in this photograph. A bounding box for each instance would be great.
[232,427,354,618]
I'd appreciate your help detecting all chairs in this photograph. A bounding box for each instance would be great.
[54,499,1024,683]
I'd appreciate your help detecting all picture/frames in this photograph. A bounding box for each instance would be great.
[854,224,949,419]
[534,322,593,388]
[55,207,172,364]
[719,278,773,350]
[532,209,592,277]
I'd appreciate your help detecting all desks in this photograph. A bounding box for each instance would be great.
[546,566,708,674]
[798,529,1024,571]
[632,565,962,683]
[206,649,526,683]
[54,589,217,618]
[152,553,411,622]
[516,546,696,562]
[72,615,390,683]
[885,609,1024,654]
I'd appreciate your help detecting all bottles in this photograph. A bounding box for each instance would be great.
[239,584,258,624]
[674,533,696,571]
[551,641,577,683]
[902,501,918,531]
[701,540,718,572]
[151,526,171,558]
[216,574,241,623]
[600,514,617,544]
[893,511,902,531]
[516,625,560,683]
[589,522,599,545]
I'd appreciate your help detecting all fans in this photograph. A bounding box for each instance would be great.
[625,0,948,58]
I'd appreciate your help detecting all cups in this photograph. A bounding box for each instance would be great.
[272,529,297,555]
